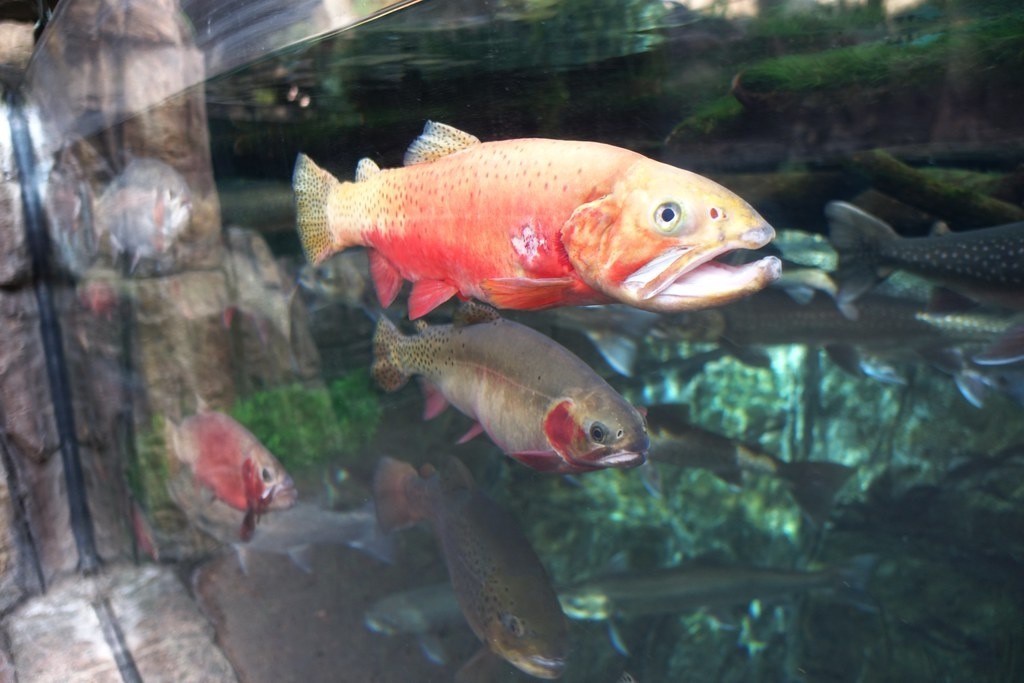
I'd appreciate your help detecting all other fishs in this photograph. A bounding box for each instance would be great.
[83,117,1023,683]
